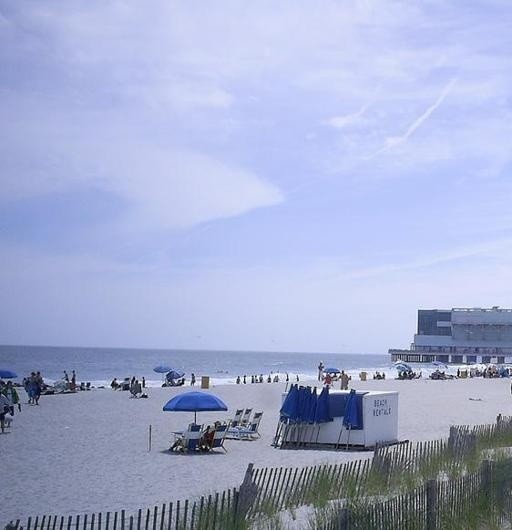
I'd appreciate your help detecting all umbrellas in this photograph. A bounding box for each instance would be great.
[152,366,170,383]
[162,390,229,425]
[271,383,362,451]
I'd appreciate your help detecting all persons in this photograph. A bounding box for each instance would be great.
[370,359,512,379]
[198,420,221,452]
[169,422,194,454]
[1,369,43,435]
[61,369,300,398]
[317,362,349,389]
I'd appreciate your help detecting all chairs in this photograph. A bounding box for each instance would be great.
[133,383,142,398]
[170,408,264,455]
